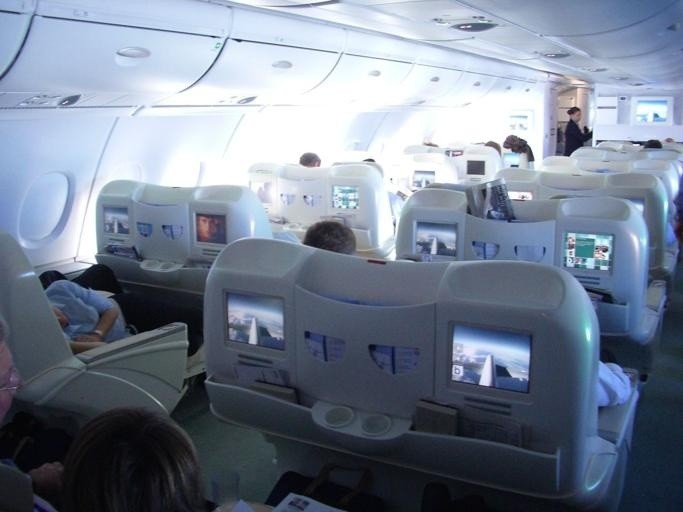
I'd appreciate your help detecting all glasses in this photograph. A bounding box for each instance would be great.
[0,374,23,391]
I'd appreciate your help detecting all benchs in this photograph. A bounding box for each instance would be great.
[572,145,682,181]
[395,188,666,374]
[544,154,682,233]
[342,149,460,209]
[95,182,270,329]
[410,144,501,198]
[201,236,637,511]
[503,152,528,170]
[597,138,683,157]
[491,168,680,298]
[1,230,190,423]
[249,160,404,258]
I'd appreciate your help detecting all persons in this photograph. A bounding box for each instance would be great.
[564,106,592,156]
[485,141,502,157]
[592,335,632,411]
[643,139,662,149]
[298,221,356,257]
[503,135,537,164]
[363,157,403,233]
[299,153,322,169]
[195,216,218,244]
[64,407,274,511]
[42,280,204,358]
[0,318,63,512]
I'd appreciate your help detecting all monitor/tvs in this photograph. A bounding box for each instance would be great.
[332,184,362,210]
[191,211,227,246]
[101,206,131,236]
[413,220,460,260]
[560,231,616,273]
[466,159,486,175]
[444,321,538,404]
[222,288,290,360]
[508,190,534,204]
[412,170,436,189]
